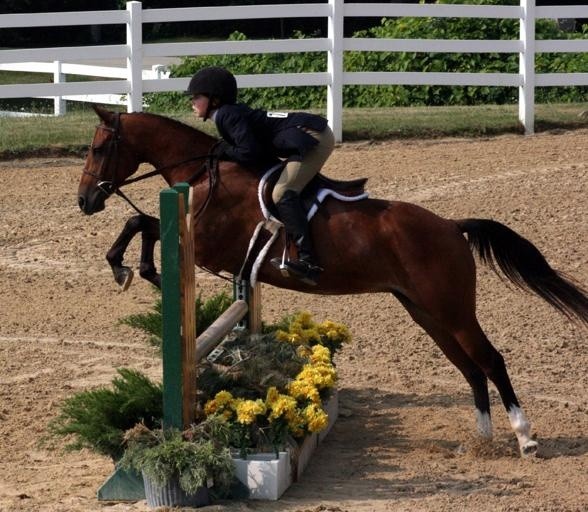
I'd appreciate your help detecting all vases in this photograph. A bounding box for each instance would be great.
[224,430,318,501]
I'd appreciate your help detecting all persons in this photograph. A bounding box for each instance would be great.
[180,67,335,285]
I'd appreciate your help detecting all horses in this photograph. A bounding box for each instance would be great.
[77,103,588,457]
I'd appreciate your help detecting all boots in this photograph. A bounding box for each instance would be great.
[269,189,322,287]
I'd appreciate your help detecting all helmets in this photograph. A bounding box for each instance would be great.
[181,65,238,108]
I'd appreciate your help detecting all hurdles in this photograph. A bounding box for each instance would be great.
[97,182,262,503]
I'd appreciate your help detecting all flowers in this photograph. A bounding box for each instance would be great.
[197,306,354,462]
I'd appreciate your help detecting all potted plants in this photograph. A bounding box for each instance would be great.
[118,417,237,507]
